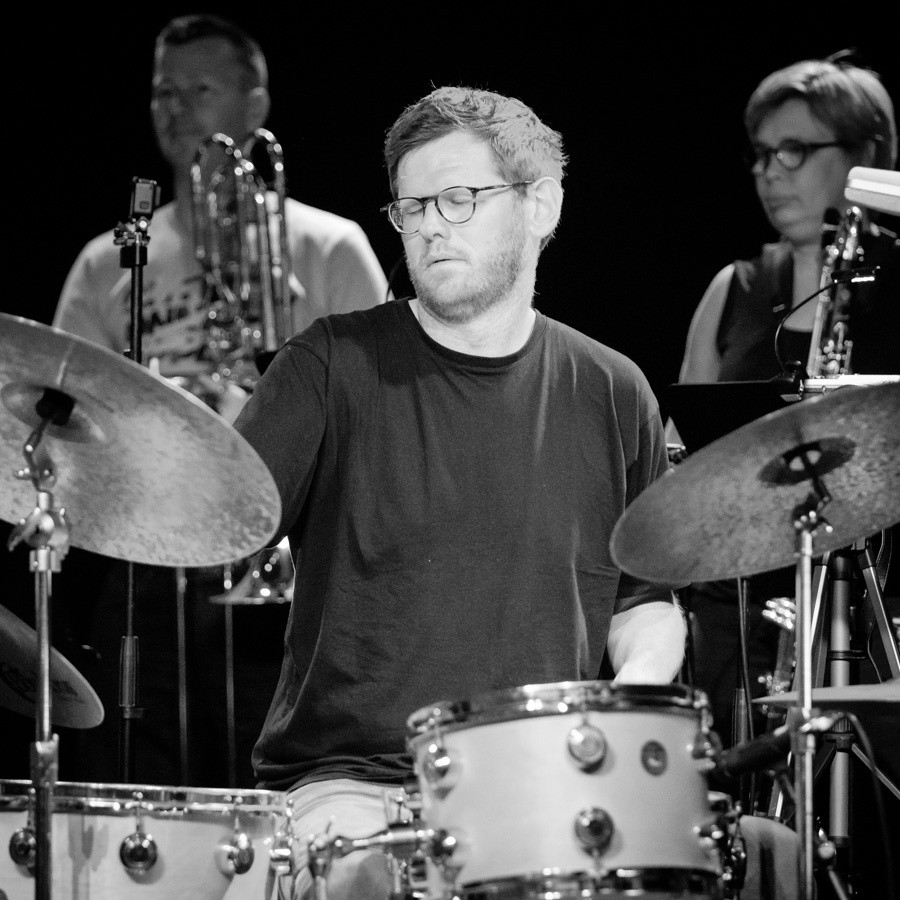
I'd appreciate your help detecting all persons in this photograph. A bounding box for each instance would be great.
[665,50,900,898]
[229,86,686,900]
[49,16,393,788]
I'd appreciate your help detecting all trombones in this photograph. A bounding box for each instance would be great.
[186,121,299,608]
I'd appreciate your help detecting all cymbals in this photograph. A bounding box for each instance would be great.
[0,311,281,568]
[611,381,899,585]
[0,605,105,732]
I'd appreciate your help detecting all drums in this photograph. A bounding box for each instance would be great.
[0,775,290,900]
[405,682,730,899]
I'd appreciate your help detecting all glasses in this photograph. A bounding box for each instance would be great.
[752,137,846,175]
[380,180,536,235]
